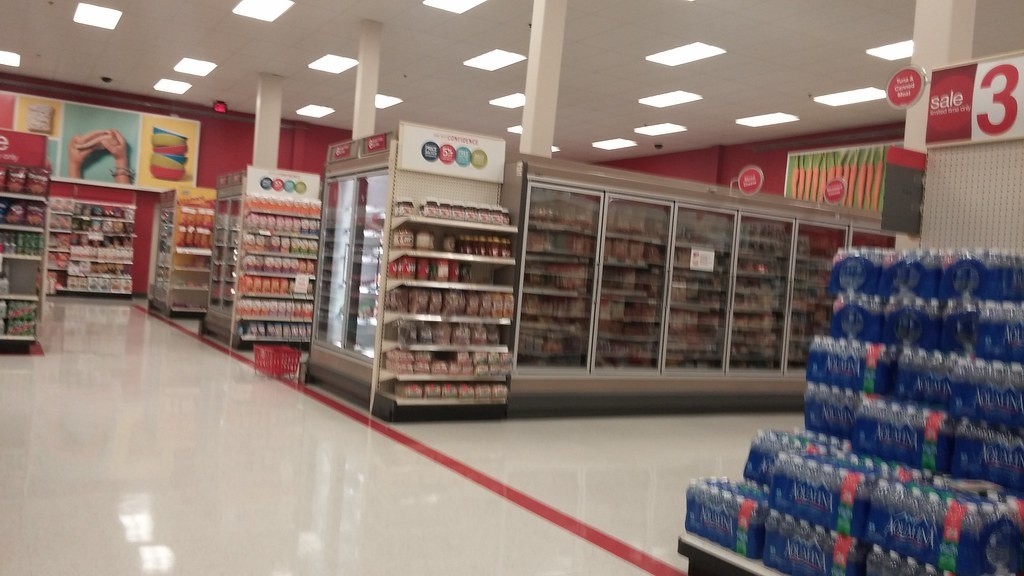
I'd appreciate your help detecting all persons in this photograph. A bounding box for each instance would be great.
[68,129,131,184]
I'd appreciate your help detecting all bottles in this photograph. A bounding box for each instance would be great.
[684,246,1024,576]
[459,233,514,258]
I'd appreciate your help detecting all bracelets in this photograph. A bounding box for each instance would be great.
[110,167,135,179]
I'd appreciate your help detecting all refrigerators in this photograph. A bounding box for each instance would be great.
[306,130,502,405]
[152,187,215,311]
[205,171,244,350]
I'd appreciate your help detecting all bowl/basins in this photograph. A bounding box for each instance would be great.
[149,126,189,180]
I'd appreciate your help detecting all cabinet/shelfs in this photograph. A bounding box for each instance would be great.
[0,191,50,357]
[46,197,135,295]
[370,174,899,423]
[146,195,321,348]
